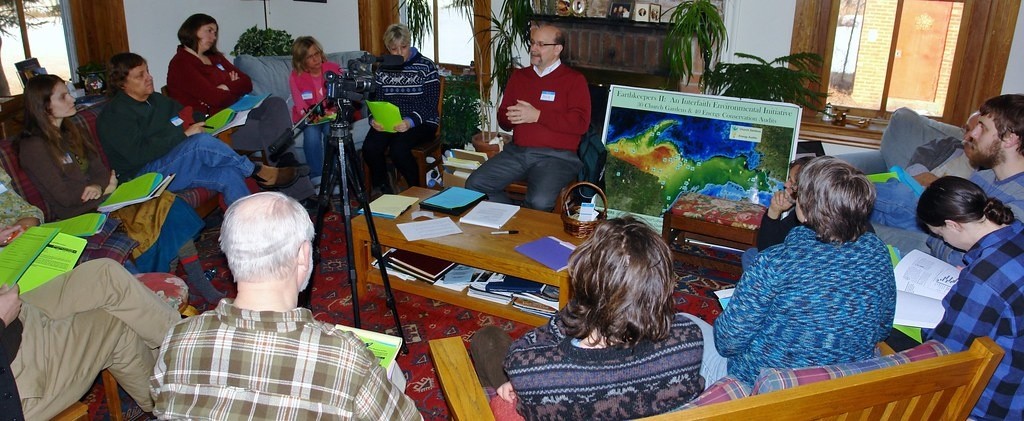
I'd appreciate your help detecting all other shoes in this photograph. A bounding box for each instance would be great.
[314,184,348,196]
[276,159,314,177]
[256,165,301,190]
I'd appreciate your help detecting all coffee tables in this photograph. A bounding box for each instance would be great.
[349,186,606,330]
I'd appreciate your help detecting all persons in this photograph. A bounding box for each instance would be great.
[95,13,348,213]
[470,214,706,421]
[613,6,630,17]
[914,92,1024,421]
[674,156,896,392]
[0,166,190,421]
[362,23,441,194]
[17,74,226,305]
[639,8,659,21]
[147,191,424,421]
[466,26,591,213]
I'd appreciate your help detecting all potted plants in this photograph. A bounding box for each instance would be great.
[392,0,535,159]
[76,61,108,94]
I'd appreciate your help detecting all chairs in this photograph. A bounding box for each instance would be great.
[161,83,291,181]
[361,77,445,201]
[49,401,90,421]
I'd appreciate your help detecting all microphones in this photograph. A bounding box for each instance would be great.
[360,54,405,65]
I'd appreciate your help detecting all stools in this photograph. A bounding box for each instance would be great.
[498,173,574,215]
[133,273,200,317]
[662,192,766,276]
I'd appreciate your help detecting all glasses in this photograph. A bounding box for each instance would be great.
[960,124,970,135]
[391,45,408,53]
[306,51,320,61]
[528,40,559,48]
[790,182,798,193]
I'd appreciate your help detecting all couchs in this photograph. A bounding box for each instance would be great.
[832,106,964,255]
[0,95,259,268]
[234,50,373,165]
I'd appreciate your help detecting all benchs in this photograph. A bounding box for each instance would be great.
[429,295,1005,421]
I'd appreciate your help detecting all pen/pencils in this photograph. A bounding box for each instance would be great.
[4,225,29,243]
[189,123,214,129]
[303,107,311,119]
[491,231,518,235]
[116,174,120,178]
[565,202,570,216]
[364,343,373,347]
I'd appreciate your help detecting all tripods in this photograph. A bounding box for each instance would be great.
[267,89,409,356]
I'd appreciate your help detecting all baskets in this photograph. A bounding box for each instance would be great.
[560,180,609,239]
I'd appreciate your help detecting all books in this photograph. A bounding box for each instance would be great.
[372,235,580,318]
[443,149,489,180]
[335,323,403,381]
[359,185,521,244]
[97,172,176,212]
[198,108,234,134]
[212,94,271,137]
[14,57,48,90]
[0,211,111,296]
[892,248,962,329]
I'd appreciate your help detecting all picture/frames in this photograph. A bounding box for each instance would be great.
[15,57,41,86]
[556,0,662,23]
